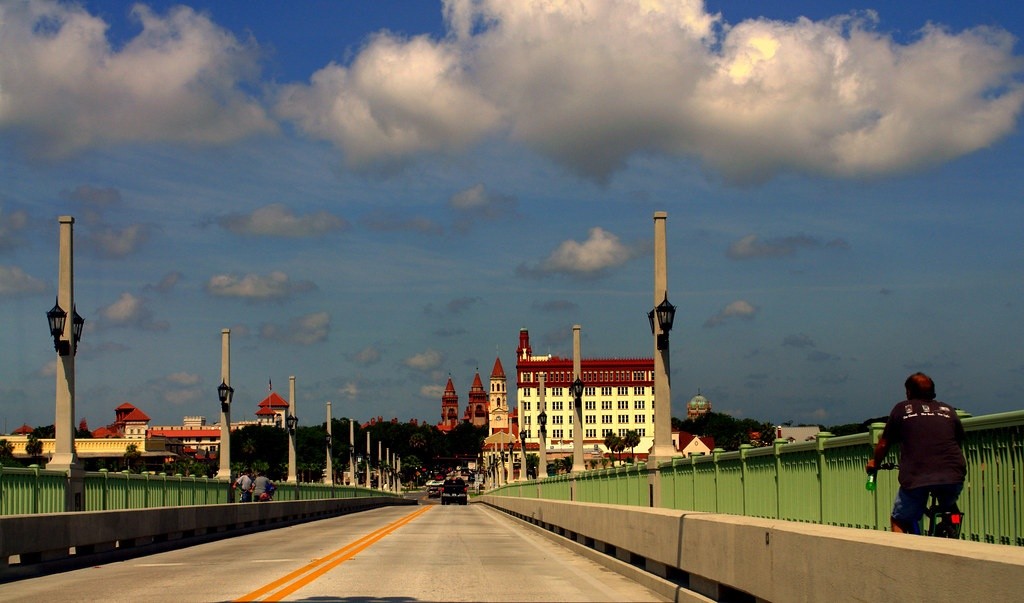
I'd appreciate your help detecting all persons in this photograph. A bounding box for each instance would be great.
[251,472,271,502]
[233,472,254,492]
[866,371,968,535]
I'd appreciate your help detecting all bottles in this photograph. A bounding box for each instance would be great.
[865,471,877,490]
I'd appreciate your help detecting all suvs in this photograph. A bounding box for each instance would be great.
[426,477,470,505]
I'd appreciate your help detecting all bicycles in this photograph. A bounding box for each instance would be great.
[237,485,278,502]
[868,463,965,540]
